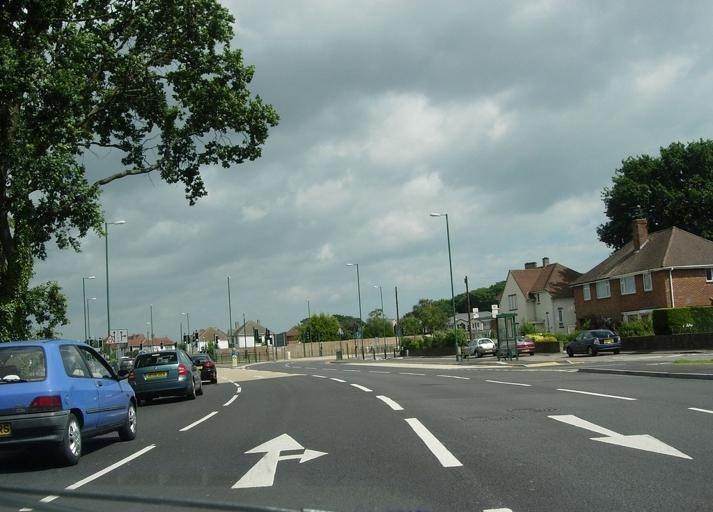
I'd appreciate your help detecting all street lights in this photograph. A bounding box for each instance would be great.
[86,296,98,346]
[428,211,461,361]
[103,218,126,341]
[373,284,387,359]
[344,263,361,359]
[144,274,246,351]
[305,298,313,356]
[81,275,96,345]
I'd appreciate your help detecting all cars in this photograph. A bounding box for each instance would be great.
[0,340,140,461]
[499,335,536,356]
[109,350,216,403]
[563,328,622,358]
[461,336,497,360]
